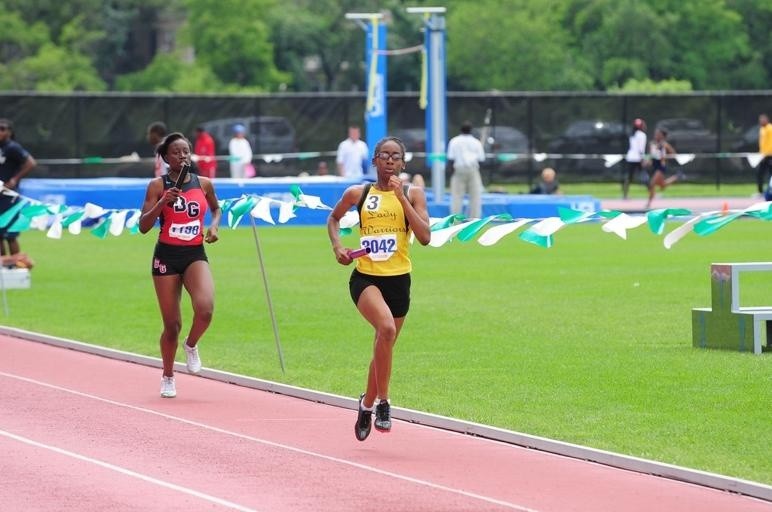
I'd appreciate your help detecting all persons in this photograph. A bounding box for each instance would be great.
[529,168,561,194]
[642,128,687,210]
[752,113,772,197]
[336,125,369,177]
[327,136,431,441]
[146,121,200,178]
[447,121,485,219]
[229,124,254,178]
[399,172,425,188]
[139,133,222,398]
[193,124,216,177]
[622,118,649,200]
[317,161,329,176]
[0,118,36,268]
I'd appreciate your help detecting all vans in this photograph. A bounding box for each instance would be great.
[183,111,300,159]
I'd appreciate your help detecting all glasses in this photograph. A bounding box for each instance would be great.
[373,150,402,162]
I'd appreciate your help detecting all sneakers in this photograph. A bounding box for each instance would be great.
[374,398,393,433]
[158,374,177,398]
[354,392,374,441]
[181,336,203,375]
